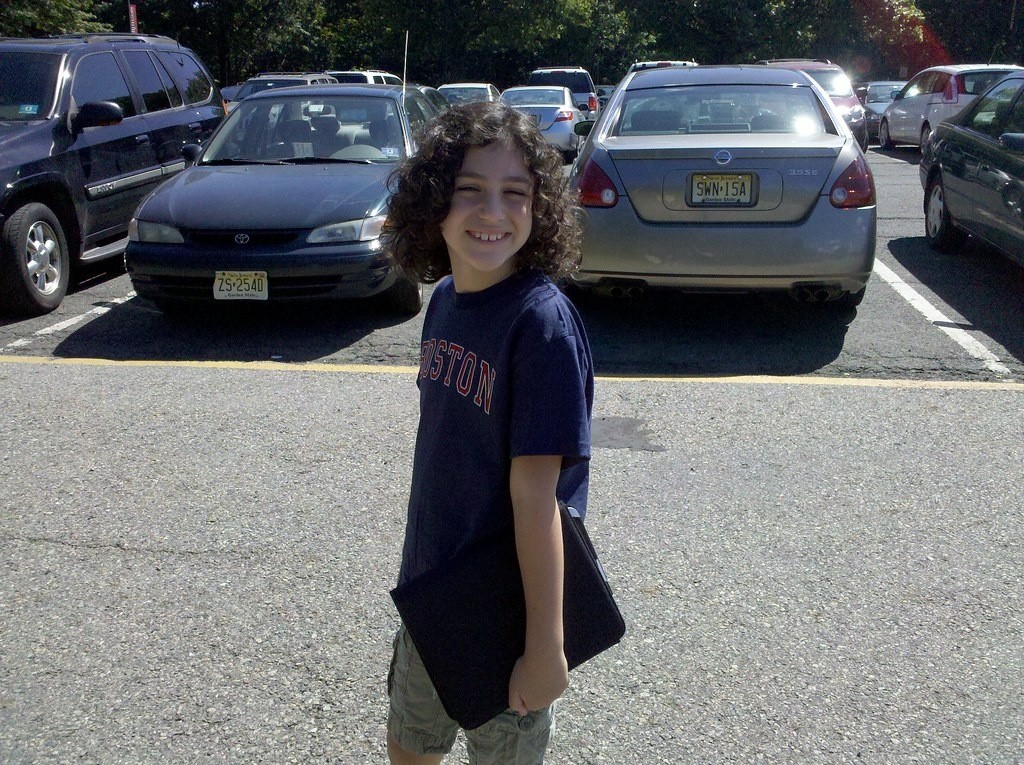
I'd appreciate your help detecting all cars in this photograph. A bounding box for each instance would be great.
[550,65,877,309]
[858,81,923,142]
[917,73,1024,264]
[594,85,616,109]
[436,83,501,123]
[498,86,589,157]
[125,84,441,316]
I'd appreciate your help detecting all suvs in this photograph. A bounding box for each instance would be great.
[0,34,229,314]
[217,68,441,139]
[621,58,700,117]
[528,64,606,130]
[755,57,871,156]
[878,62,1024,150]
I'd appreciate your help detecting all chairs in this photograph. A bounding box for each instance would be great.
[991,100,1024,128]
[973,81,986,94]
[266,117,389,157]
[448,95,457,103]
[751,115,786,129]
[631,111,679,130]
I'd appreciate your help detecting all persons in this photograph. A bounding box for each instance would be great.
[381,100,593,764]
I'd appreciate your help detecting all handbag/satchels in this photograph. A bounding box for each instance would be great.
[390,499,626,730]
